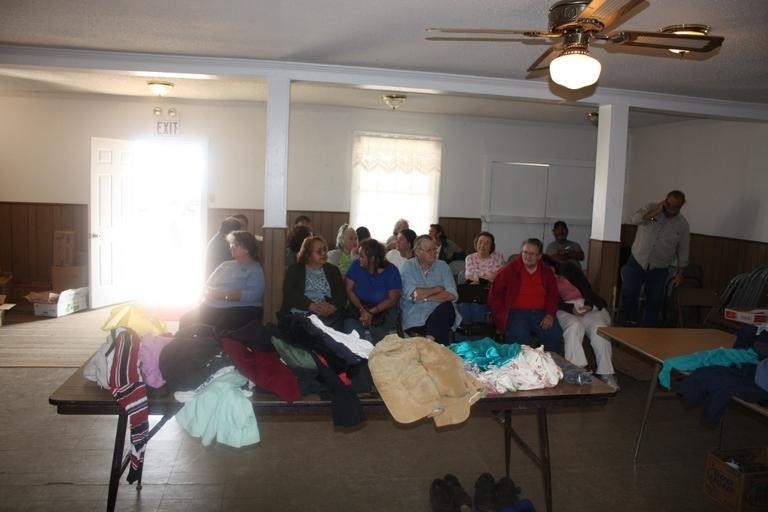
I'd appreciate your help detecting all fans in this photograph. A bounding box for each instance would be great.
[425,0,724,71]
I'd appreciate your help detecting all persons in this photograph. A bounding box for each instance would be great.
[181,213,400,347]
[539,254,621,395]
[545,219,584,271]
[396,229,418,260]
[383,219,409,254]
[456,231,505,305]
[621,190,691,329]
[429,223,466,265]
[486,237,565,360]
[398,234,464,349]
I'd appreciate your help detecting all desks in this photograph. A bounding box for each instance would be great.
[48,348,616,512]
[597,327,768,460]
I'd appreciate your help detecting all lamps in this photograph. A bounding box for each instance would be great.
[588,111,599,121]
[148,81,174,98]
[549,27,602,90]
[382,96,407,108]
[153,106,176,117]
[658,25,710,54]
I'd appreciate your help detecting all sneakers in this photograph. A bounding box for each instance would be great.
[430,478,456,511]
[497,477,521,511]
[476,473,497,512]
[443,474,471,512]
[600,374,622,392]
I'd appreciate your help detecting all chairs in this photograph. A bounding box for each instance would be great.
[456,284,497,337]
[677,289,721,328]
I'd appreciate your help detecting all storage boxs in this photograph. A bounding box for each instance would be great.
[703,447,768,512]
[0,232,88,328]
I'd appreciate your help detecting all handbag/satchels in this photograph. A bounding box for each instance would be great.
[455,277,491,305]
[344,299,383,326]
[303,295,344,337]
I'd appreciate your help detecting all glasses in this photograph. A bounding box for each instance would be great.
[312,248,328,257]
[419,248,439,253]
